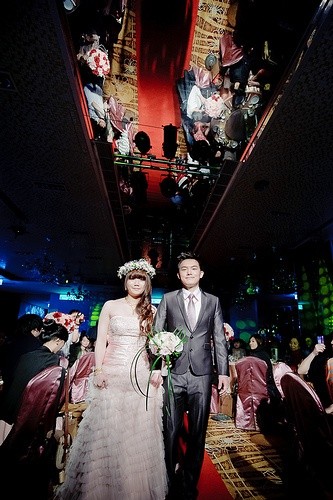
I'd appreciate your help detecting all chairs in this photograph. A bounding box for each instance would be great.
[210,356,333,470]
[0,353,95,462]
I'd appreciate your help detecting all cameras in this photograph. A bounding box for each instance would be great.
[317,335,324,345]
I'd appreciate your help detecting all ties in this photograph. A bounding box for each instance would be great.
[187,295,198,328]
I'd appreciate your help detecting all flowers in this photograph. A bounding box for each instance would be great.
[131,325,187,412]
[78,45,110,77]
[222,323,235,342]
[42,312,75,334]
[117,258,156,279]
[204,93,224,118]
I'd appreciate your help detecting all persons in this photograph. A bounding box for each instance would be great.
[226,333,333,417]
[0,310,93,500]
[74,258,179,500]
[150,252,227,500]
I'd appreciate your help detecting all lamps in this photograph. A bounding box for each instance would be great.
[160,172,179,199]
[129,170,148,201]
[133,132,153,155]
[162,125,179,160]
